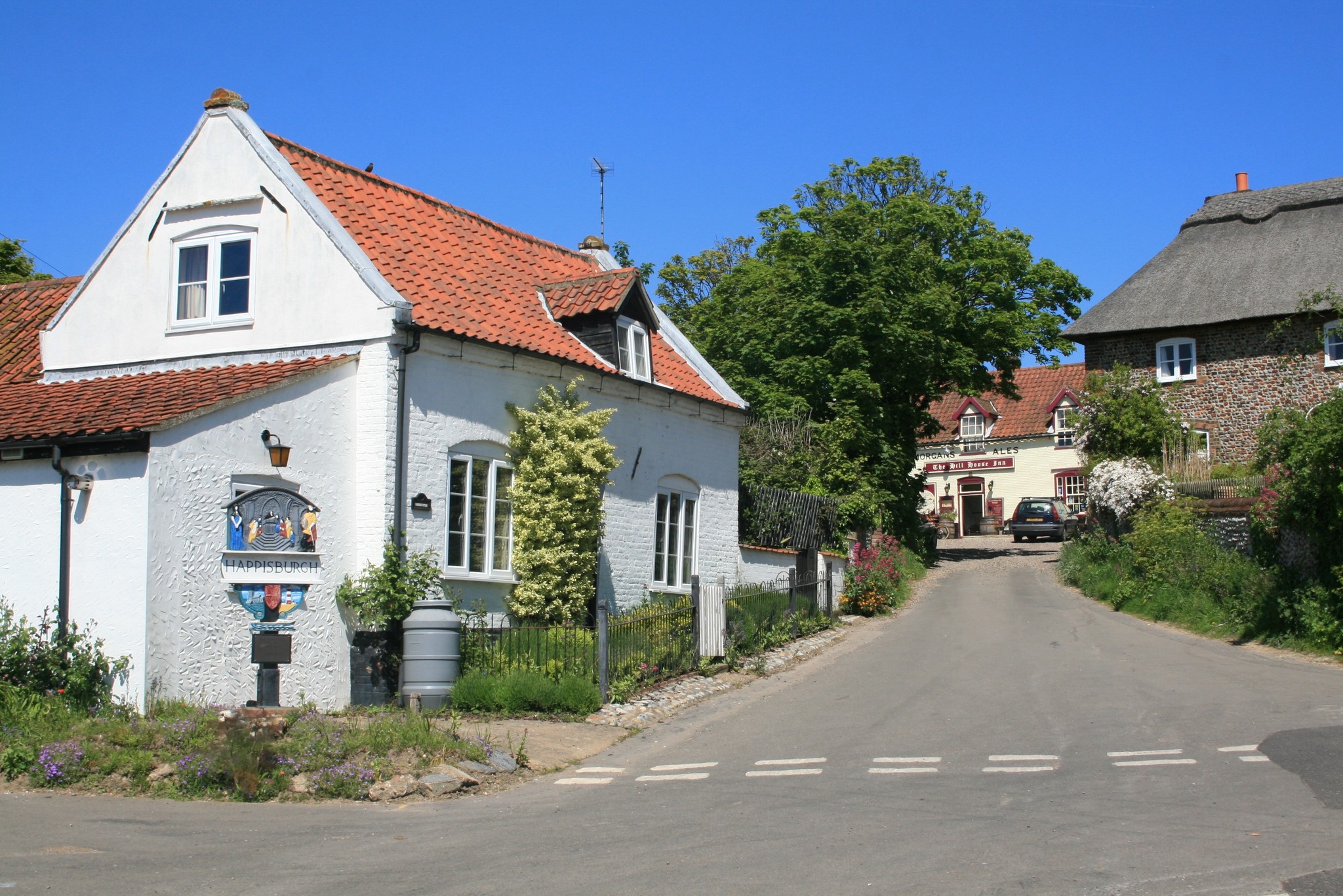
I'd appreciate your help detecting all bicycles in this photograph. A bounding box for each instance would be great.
[933,519,950,539]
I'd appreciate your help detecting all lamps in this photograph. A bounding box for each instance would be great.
[944,483,951,493]
[988,481,994,492]
[261,430,291,468]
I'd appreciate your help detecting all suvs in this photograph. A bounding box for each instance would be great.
[1010,496,1080,542]
